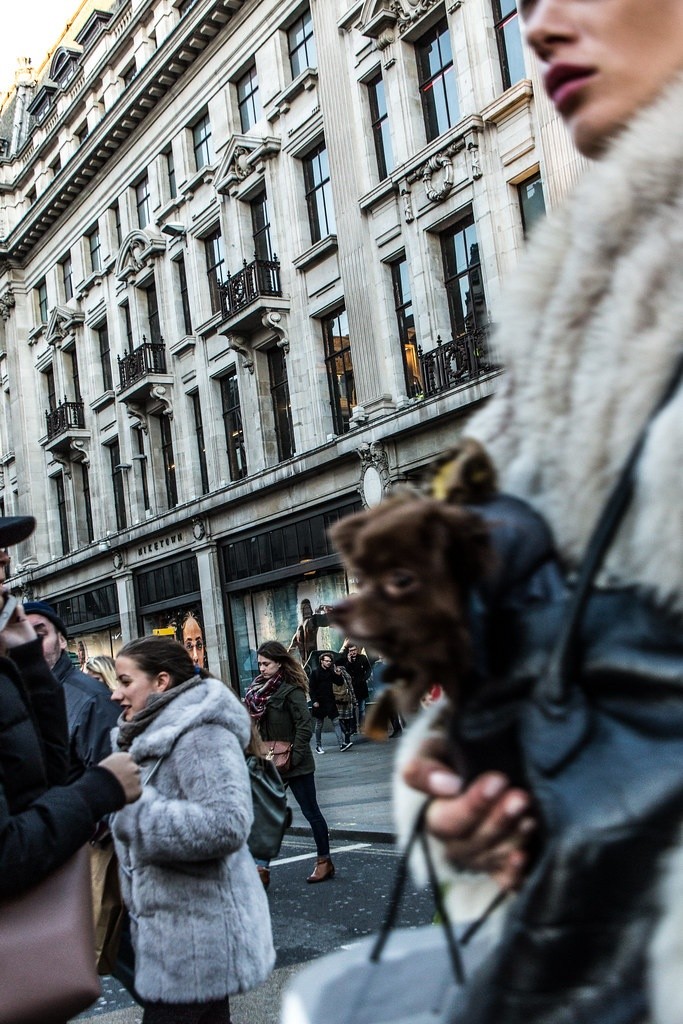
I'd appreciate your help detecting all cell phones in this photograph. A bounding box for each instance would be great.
[0,595,16,631]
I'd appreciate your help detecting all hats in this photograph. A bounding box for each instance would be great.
[23,602,67,654]
[0,517,36,548]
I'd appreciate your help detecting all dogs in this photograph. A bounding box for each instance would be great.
[327,435,683,1023]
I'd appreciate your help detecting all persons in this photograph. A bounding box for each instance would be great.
[175,609,206,671]
[308,643,407,754]
[0,516,276,1024]
[77,640,89,674]
[402,0,683,1024]
[244,641,335,888]
[287,599,334,667]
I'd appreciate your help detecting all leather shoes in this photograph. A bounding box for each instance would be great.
[306,858,335,882]
[258,868,270,891]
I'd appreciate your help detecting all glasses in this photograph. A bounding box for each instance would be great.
[323,660,331,663]
[349,649,357,652]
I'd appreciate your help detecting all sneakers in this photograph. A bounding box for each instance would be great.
[340,742,353,752]
[315,746,325,754]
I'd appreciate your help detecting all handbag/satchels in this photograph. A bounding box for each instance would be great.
[90,831,123,976]
[0,843,101,1023]
[333,670,351,710]
[280,794,511,1024]
[245,741,293,779]
[245,755,292,861]
[507,356,683,938]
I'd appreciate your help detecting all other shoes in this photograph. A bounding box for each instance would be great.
[389,727,402,738]
